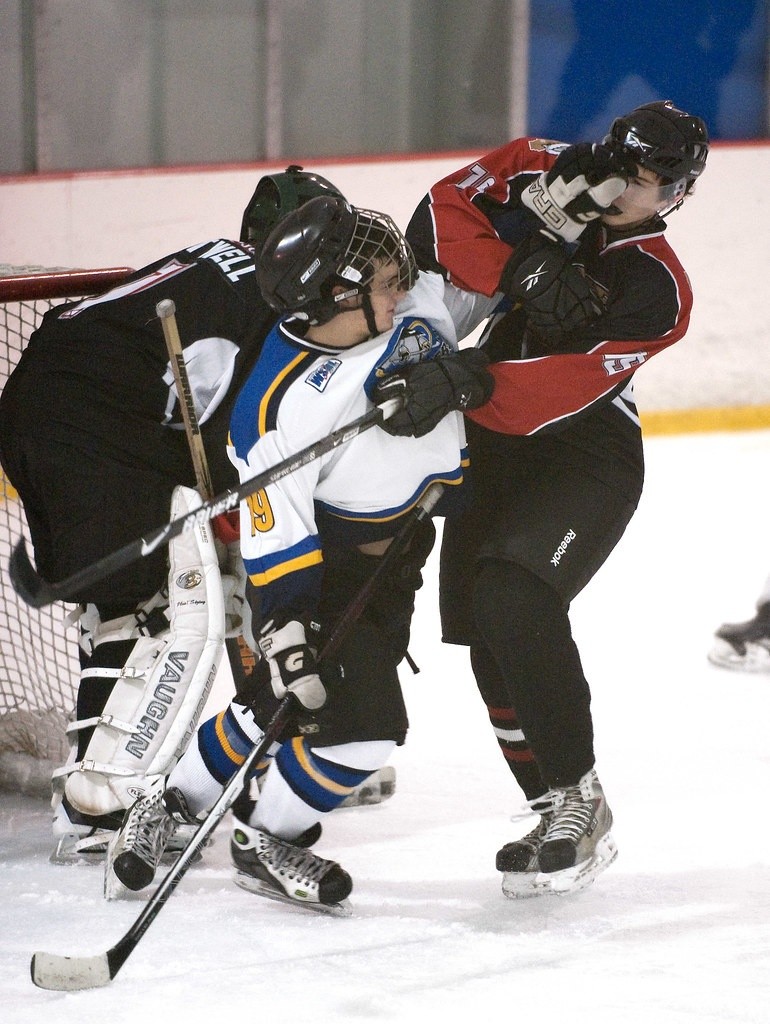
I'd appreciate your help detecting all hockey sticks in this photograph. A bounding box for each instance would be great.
[155,298,398,811]
[5,396,399,609]
[28,484,447,995]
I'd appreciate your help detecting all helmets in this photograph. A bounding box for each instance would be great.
[241,165,345,243]
[256,196,418,311]
[609,101,708,177]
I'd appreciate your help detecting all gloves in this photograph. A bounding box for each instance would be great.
[521,142,637,248]
[252,600,343,717]
[510,247,607,343]
[374,348,493,438]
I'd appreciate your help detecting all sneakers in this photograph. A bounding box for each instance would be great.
[51,793,217,868]
[521,769,619,894]
[247,768,396,810]
[495,806,554,899]
[104,776,203,900]
[705,601,770,672]
[231,801,354,917]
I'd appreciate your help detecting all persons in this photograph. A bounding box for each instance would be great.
[0,167,351,866]
[104,139,639,918]
[406,98,710,898]
